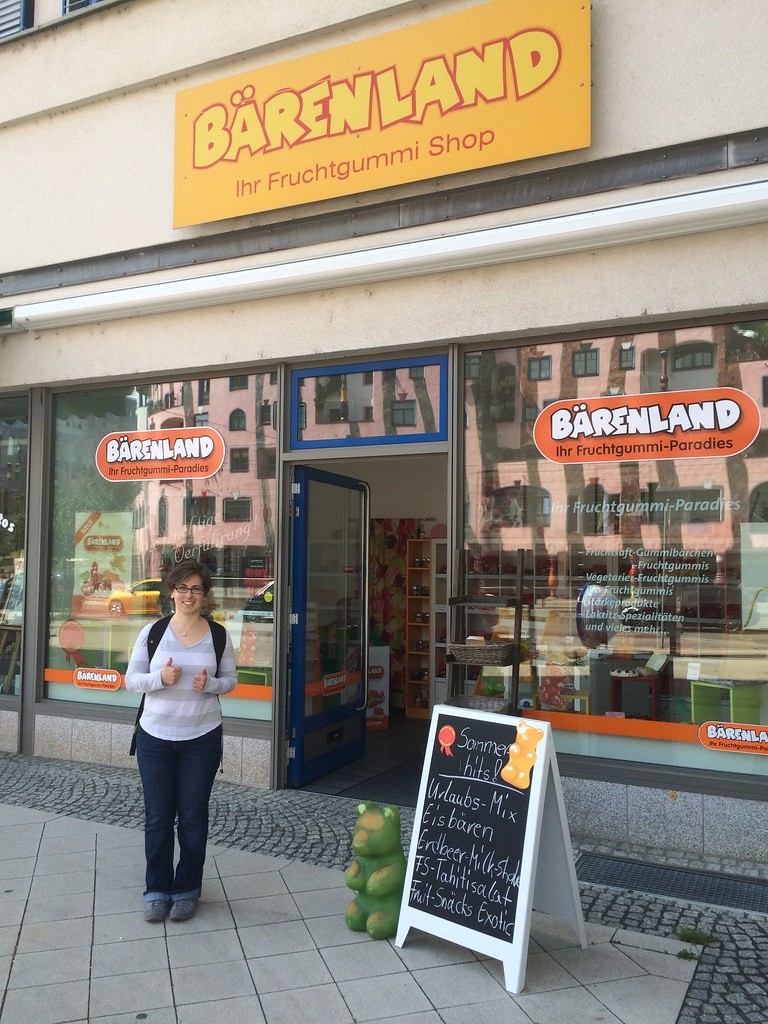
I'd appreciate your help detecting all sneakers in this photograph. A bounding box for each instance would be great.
[143,900,172,921]
[170,898,200,920]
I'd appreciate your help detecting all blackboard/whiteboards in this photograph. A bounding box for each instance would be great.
[397,705,550,993]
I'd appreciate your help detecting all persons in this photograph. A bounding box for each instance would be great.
[124,559,239,921]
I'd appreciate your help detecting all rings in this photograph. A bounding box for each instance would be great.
[194,683,197,687]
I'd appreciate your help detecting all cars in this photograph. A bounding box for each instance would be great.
[0,570,73,612]
[242,581,274,623]
[621,584,742,634]
[105,577,216,619]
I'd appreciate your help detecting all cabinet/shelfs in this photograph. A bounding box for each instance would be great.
[405,538,768,729]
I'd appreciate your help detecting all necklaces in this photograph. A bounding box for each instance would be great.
[173,616,200,636]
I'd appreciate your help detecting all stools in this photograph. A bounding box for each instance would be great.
[532,688,593,715]
[610,671,669,721]
[690,680,760,725]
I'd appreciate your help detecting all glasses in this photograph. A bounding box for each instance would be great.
[174,583,205,594]
